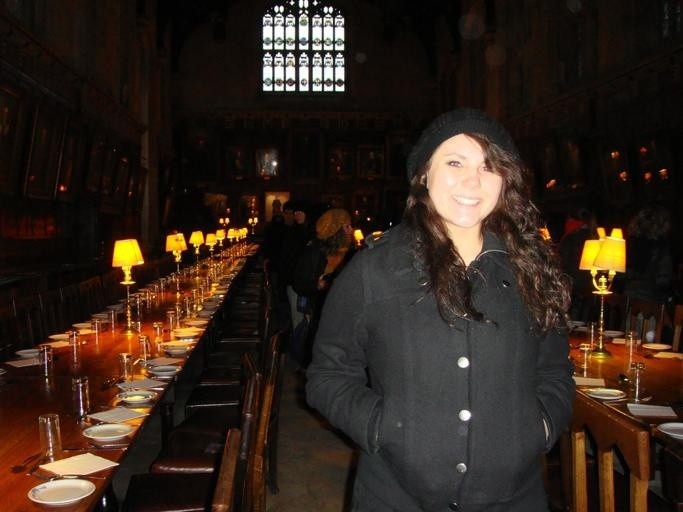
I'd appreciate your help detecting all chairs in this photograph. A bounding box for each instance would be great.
[530,284,682,511]
[0,226,282,510]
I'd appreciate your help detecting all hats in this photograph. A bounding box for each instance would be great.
[407,106,523,178]
[315,208,348,240]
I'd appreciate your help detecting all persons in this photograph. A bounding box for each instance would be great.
[304,108,576,512]
[264,201,358,378]
[559,206,673,342]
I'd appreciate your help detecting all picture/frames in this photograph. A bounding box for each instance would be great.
[0,86,23,196]
[21,96,70,203]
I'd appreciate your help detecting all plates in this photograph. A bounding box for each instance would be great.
[564,314,674,405]
[1,238,260,481]
[27,479,97,506]
[658,422,683,440]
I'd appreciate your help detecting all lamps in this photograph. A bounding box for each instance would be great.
[578,221,630,363]
